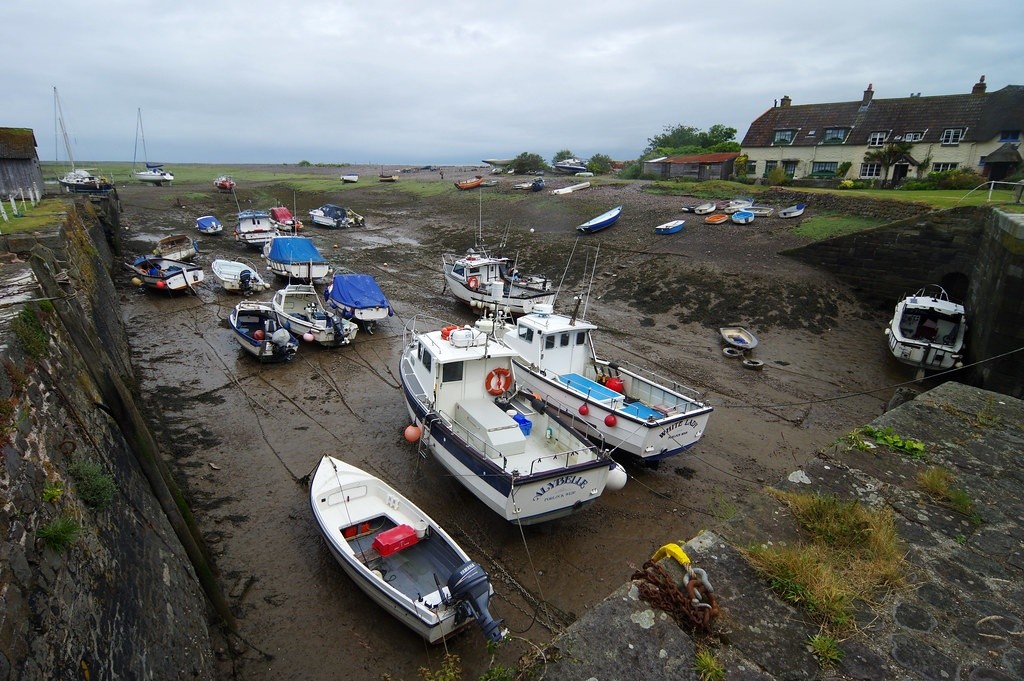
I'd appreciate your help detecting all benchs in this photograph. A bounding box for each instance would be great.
[355,535,428,563]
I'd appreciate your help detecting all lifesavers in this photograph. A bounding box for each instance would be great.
[466,257,481,261]
[441,326,464,335]
[140,269,148,275]
[485,368,511,397]
[723,347,743,359]
[743,359,763,370]
[468,277,478,289]
[441,334,448,340]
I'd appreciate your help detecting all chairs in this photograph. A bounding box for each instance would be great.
[265,319,278,338]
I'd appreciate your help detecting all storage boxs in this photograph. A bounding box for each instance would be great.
[513,413,532,436]
[372,524,417,556]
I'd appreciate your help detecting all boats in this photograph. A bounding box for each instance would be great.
[472,236,714,470]
[309,452,505,646]
[554,158,588,174]
[777,203,809,219]
[272,251,360,348]
[694,201,716,215]
[440,240,559,318]
[123,186,366,298]
[512,176,546,193]
[398,293,628,529]
[212,174,237,193]
[322,267,394,335]
[731,211,755,225]
[885,283,969,385]
[723,197,775,217]
[378,165,399,182]
[340,173,360,183]
[655,219,686,234]
[226,298,300,364]
[453,175,484,191]
[704,213,729,225]
[575,204,624,234]
[719,327,758,351]
[479,179,498,186]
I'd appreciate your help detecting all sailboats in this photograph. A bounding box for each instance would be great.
[53,86,116,197]
[128,108,175,187]
